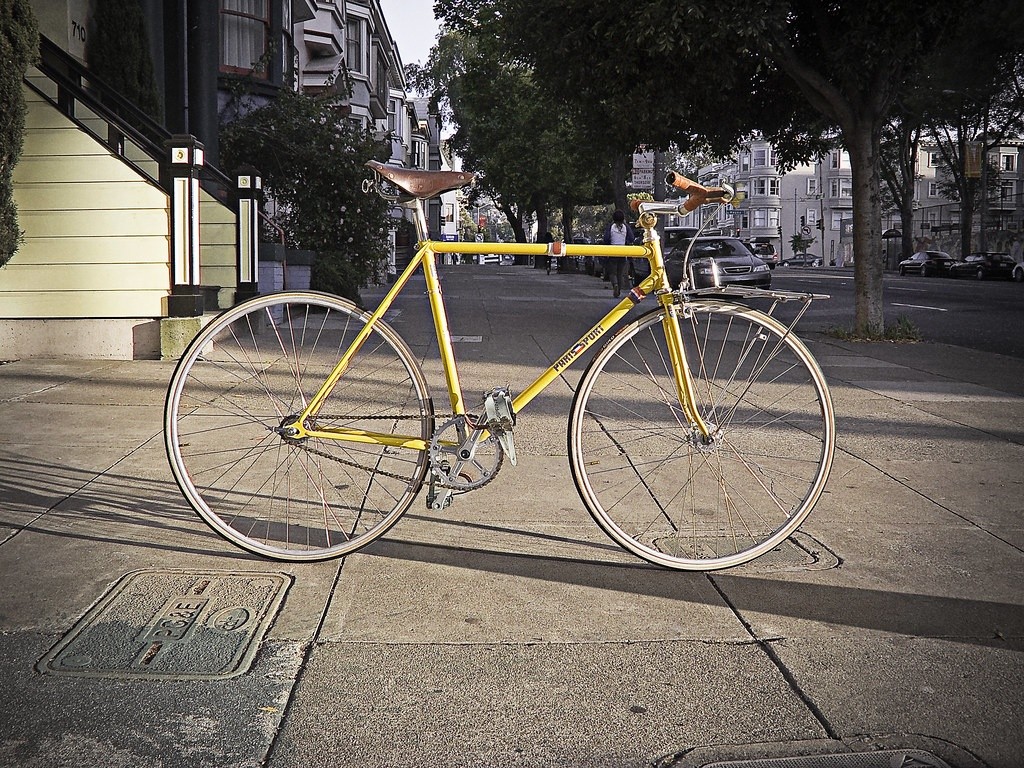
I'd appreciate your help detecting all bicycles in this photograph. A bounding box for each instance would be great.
[164,160,836,572]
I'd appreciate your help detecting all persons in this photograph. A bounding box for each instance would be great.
[452,253,458,265]
[604,209,632,296]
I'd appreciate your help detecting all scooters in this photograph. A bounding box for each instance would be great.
[545,258,552,276]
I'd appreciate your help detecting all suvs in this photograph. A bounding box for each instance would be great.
[747,238,779,269]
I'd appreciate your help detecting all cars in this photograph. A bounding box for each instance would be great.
[1012,262,1024,282]
[949,252,1017,281]
[626,227,705,286]
[776,253,823,267]
[558,236,610,280]
[664,236,772,298]
[898,251,952,277]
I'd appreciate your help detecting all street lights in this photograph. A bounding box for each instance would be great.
[737,227,740,237]
[478,201,498,225]
[814,159,825,266]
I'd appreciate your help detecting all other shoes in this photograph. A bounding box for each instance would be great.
[613,284,620,298]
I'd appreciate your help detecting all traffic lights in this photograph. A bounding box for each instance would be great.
[816,219,822,230]
[777,226,782,234]
[800,216,804,226]
